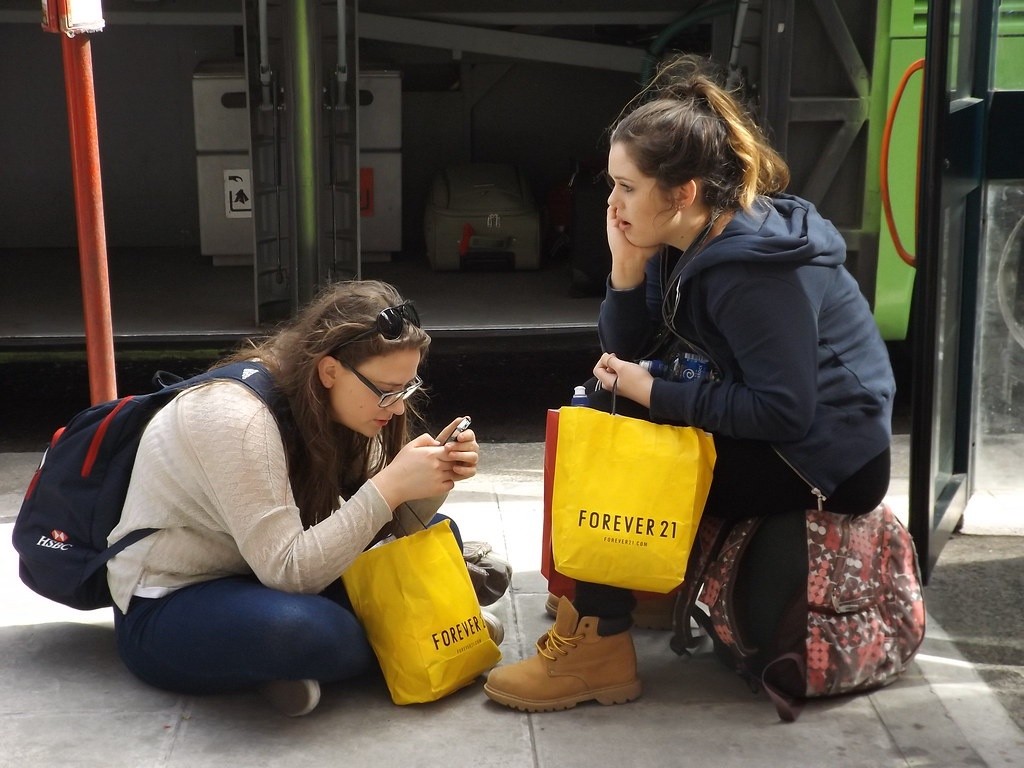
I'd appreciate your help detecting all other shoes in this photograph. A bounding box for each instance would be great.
[261,676,322,718]
[477,609,505,645]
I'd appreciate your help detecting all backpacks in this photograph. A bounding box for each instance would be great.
[12,359,307,611]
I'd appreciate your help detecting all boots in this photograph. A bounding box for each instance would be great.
[545,588,678,631]
[483,594,642,713]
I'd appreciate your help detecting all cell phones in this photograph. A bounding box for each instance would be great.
[443,418,471,446]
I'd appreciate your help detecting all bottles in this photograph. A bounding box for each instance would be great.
[571,386,592,409]
[638,353,721,385]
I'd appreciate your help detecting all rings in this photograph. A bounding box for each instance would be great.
[474,452,479,464]
[607,356,614,368]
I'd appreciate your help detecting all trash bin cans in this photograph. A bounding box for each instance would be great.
[193,59,402,283]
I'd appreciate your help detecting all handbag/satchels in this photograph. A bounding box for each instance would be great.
[339,502,503,706]
[540,352,686,602]
[551,378,717,596]
[461,540,512,607]
[670,502,926,723]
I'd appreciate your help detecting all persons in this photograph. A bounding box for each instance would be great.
[110,281,514,718]
[483,55,896,713]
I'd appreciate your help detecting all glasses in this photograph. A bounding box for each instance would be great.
[333,301,420,350]
[329,353,423,408]
